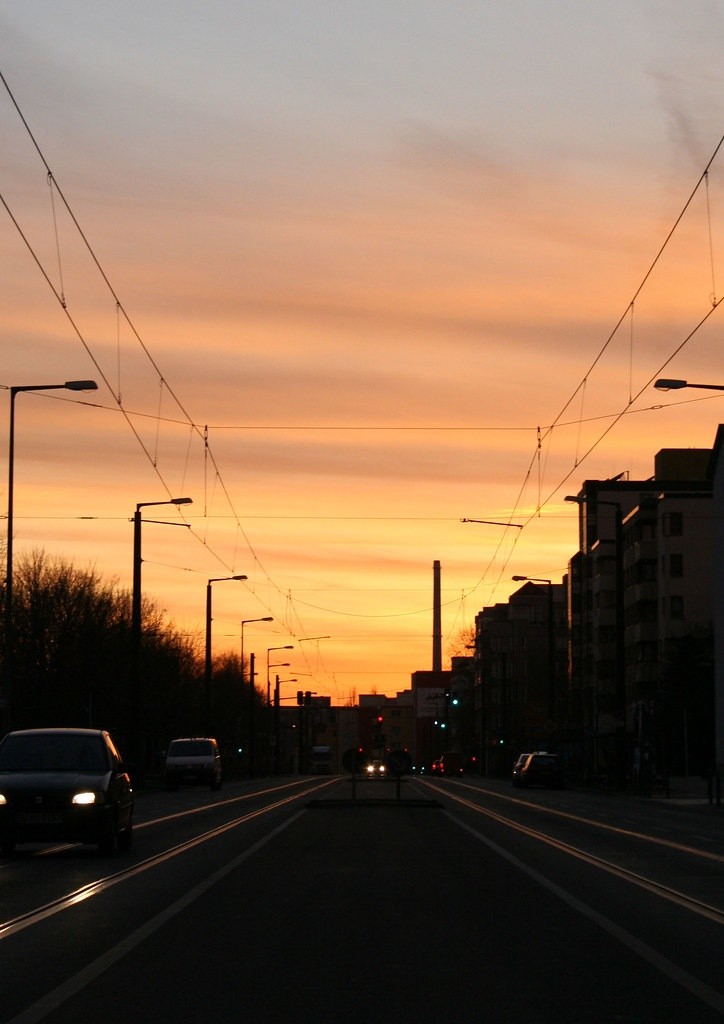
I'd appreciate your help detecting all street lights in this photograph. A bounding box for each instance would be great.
[239,616,274,724]
[3,378,99,728]
[512,576,560,774]
[128,497,197,748]
[205,574,252,738]
[265,644,297,734]
[563,493,628,794]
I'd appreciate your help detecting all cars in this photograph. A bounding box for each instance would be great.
[512,751,561,787]
[0,727,135,862]
[411,750,481,779]
[366,762,390,779]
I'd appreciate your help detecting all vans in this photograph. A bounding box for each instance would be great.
[161,738,223,794]
